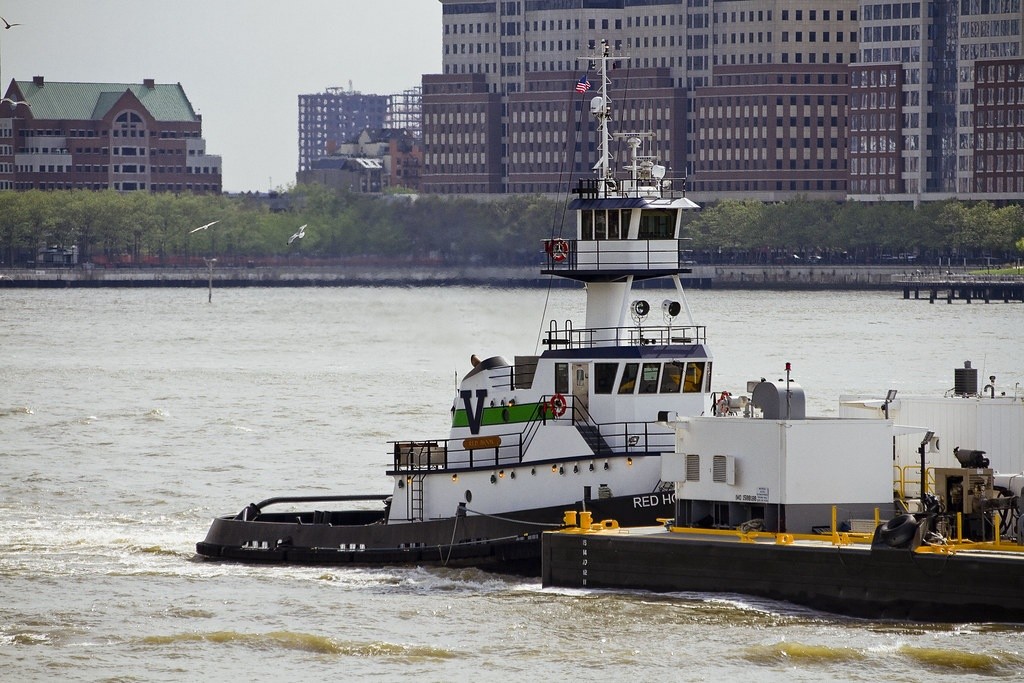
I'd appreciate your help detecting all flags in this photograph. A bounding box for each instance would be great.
[576,73,591,94]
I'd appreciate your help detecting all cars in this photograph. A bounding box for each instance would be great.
[899,252,917,262]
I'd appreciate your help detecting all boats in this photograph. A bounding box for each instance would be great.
[197,36,806,576]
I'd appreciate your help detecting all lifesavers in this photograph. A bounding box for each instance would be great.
[550,394,567,417]
[549,238,569,261]
[720,391,730,413]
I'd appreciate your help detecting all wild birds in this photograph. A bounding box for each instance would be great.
[0,17,22,30]
[187,218,221,235]
[1,97,32,111]
[285,223,308,247]
